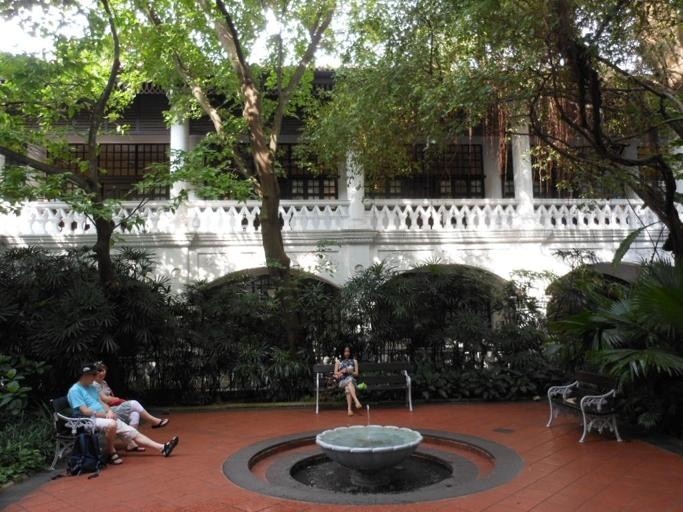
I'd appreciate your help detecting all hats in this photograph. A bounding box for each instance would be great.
[77,363,100,376]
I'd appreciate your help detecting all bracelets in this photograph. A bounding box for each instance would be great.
[352,371,355,376]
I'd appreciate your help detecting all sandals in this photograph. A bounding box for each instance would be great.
[161,435,178,456]
[108,452,123,465]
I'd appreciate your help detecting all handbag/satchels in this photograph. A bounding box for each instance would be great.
[107,399,126,406]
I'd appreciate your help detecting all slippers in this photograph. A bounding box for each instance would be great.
[151,418,169,428]
[126,444,147,452]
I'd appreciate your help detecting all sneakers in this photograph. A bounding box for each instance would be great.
[348,405,362,417]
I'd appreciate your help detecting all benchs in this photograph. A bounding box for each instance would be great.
[53,397,98,474]
[312,359,414,415]
[545,373,624,444]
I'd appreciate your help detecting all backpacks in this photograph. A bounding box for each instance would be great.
[66,431,101,477]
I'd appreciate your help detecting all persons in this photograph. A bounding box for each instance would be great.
[92,360,168,451]
[66,362,179,464]
[332,344,361,416]
